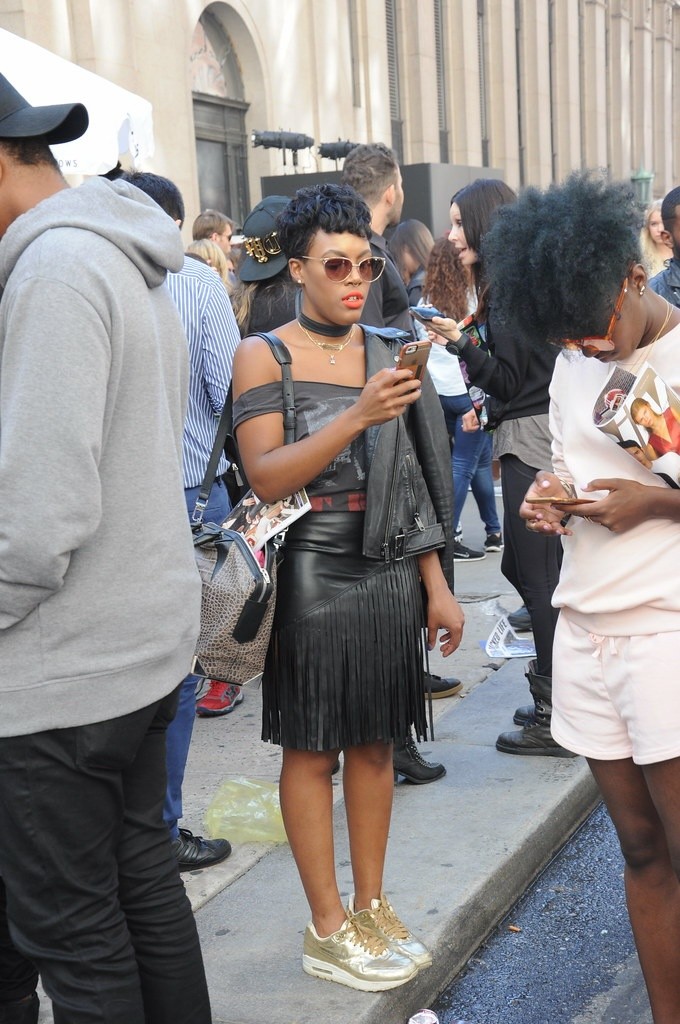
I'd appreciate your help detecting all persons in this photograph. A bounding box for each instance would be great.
[0,876,41,1024]
[631,398,680,460]
[0,75,680,874]
[604,389,627,412]
[0,75,215,1024]
[234,185,464,991]
[480,165,680,1024]
[617,439,680,488]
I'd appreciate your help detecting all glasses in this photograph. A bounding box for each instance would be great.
[219,233,232,242]
[556,260,637,352]
[301,255,386,283]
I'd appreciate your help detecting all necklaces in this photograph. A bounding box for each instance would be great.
[608,302,673,379]
[306,330,356,365]
[635,295,669,377]
[297,318,353,350]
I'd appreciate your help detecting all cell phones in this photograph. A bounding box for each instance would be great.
[526,497,598,505]
[392,340,433,397]
[409,306,445,320]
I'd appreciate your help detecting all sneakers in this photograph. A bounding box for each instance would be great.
[170,827,232,873]
[346,892,433,971]
[423,670,463,699]
[453,542,486,562]
[483,531,504,552]
[195,676,243,716]
[303,908,418,992]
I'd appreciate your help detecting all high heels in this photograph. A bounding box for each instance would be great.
[394,726,447,785]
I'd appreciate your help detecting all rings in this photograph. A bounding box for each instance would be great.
[583,516,593,523]
[532,521,537,533]
[461,425,464,427]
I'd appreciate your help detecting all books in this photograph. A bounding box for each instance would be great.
[218,479,312,555]
[592,361,680,488]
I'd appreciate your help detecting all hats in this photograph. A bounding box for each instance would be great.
[0,71,90,144]
[239,194,294,282]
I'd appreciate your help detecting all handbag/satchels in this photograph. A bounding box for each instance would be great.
[453,311,513,432]
[182,517,279,685]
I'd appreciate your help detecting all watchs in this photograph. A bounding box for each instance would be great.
[447,333,469,355]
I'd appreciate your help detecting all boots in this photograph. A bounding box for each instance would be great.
[495,656,579,758]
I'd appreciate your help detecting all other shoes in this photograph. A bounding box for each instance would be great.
[507,604,533,628]
